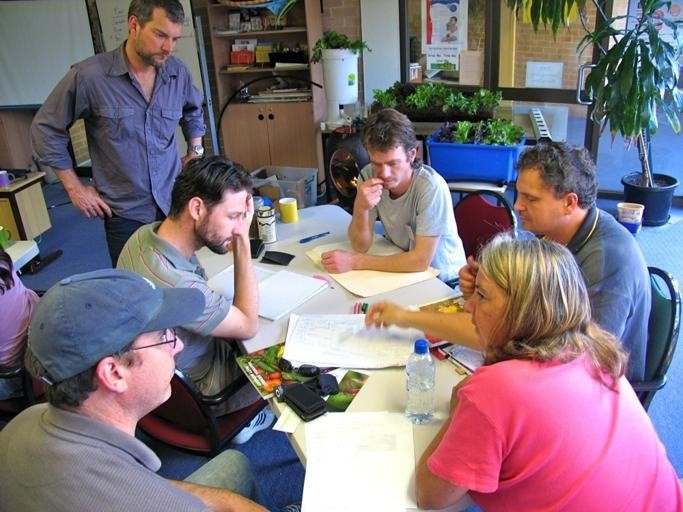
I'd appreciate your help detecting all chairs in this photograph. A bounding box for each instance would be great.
[454,189,518,264]
[628,266,683,411]
[138,367,269,457]
[0,365,48,423]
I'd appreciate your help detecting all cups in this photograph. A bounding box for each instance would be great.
[0,170,16,188]
[248,196,265,239]
[256,206,277,244]
[617,203,645,233]
[278,197,299,224]
[0,226,12,251]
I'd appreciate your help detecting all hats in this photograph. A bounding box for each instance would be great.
[18,263,212,386]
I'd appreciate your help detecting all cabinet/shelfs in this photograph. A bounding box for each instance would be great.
[190,0,330,202]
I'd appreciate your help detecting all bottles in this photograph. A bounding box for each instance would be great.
[403,337,437,427]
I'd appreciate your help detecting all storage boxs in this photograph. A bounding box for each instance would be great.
[249,165,318,210]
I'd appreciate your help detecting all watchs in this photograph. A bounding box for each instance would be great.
[186,143,204,155]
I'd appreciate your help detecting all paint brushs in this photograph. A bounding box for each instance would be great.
[353,162,377,208]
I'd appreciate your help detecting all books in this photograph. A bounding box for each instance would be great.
[233,340,343,400]
[409,292,485,378]
[247,83,313,102]
[254,267,328,323]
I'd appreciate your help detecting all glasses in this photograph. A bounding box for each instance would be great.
[122,327,178,352]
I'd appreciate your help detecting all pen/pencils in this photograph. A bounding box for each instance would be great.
[354,302,369,313]
[300,231,330,244]
[46,201,74,210]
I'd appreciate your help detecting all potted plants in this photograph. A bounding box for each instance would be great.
[312,28,371,105]
[371,80,503,123]
[505,1,683,227]
[426,118,527,183]
[440,59,459,81]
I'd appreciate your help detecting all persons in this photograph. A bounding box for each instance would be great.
[441,16,458,42]
[319,105,469,285]
[361,226,682,512]
[29,0,207,267]
[113,154,276,446]
[0,243,41,400]
[458,138,652,399]
[0,268,273,512]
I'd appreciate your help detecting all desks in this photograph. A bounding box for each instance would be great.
[320,113,536,215]
[192,204,499,511]
[0,171,63,275]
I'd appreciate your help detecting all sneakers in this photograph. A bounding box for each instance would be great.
[230,407,276,445]
